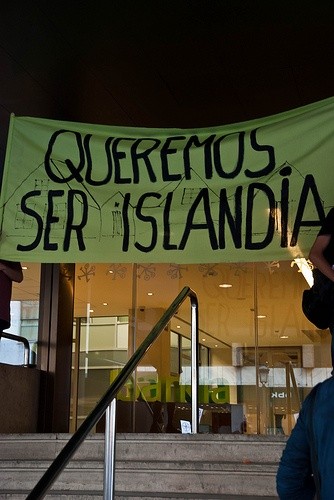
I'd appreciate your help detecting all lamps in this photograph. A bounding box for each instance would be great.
[258,363,270,384]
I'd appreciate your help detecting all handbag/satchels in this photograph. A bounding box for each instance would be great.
[303,290,330,329]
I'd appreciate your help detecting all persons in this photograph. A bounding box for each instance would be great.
[308,206,334,376]
[276,376,334,500]
[0,260,24,339]
